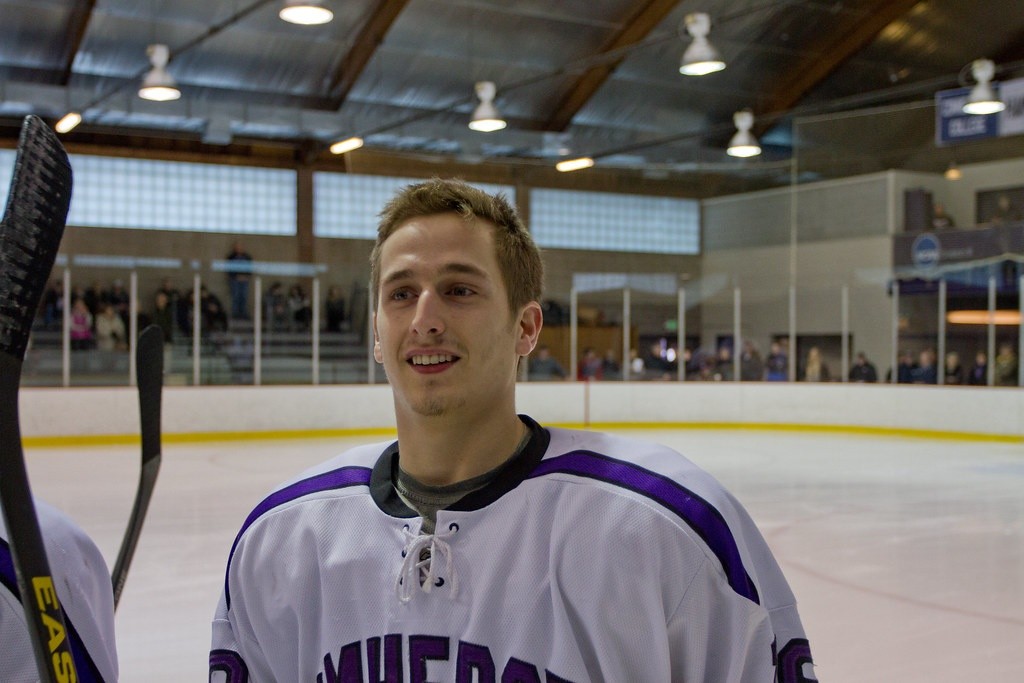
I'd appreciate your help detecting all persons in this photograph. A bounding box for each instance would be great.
[261,282,344,333]
[39,278,228,354]
[207,175,816,683]
[527,345,566,381]
[226,240,252,320]
[577,340,831,382]
[885,343,1019,387]
[849,350,877,383]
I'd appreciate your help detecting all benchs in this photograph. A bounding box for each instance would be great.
[14,319,387,385]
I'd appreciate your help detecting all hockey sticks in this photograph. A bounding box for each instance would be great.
[1,111,81,683]
[109,324,165,611]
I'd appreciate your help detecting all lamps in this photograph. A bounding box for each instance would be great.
[277,0,336,26]
[726,108,762,158]
[678,12,727,76]
[468,82,507,133]
[960,59,1007,115]
